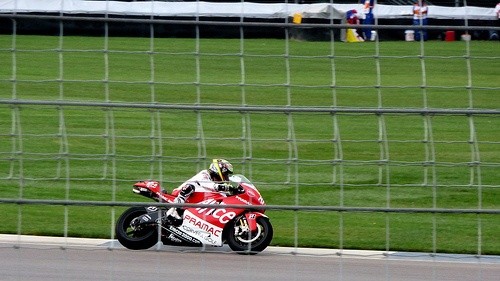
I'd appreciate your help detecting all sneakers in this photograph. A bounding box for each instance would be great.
[166,211,183,225]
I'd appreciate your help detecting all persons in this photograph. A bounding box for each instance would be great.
[165,157,243,220]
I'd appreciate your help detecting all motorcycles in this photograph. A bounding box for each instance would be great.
[114,173,273,255]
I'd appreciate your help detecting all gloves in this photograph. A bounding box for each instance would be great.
[231,187,245,194]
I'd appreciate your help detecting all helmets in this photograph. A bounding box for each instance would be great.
[208,158,234,179]
